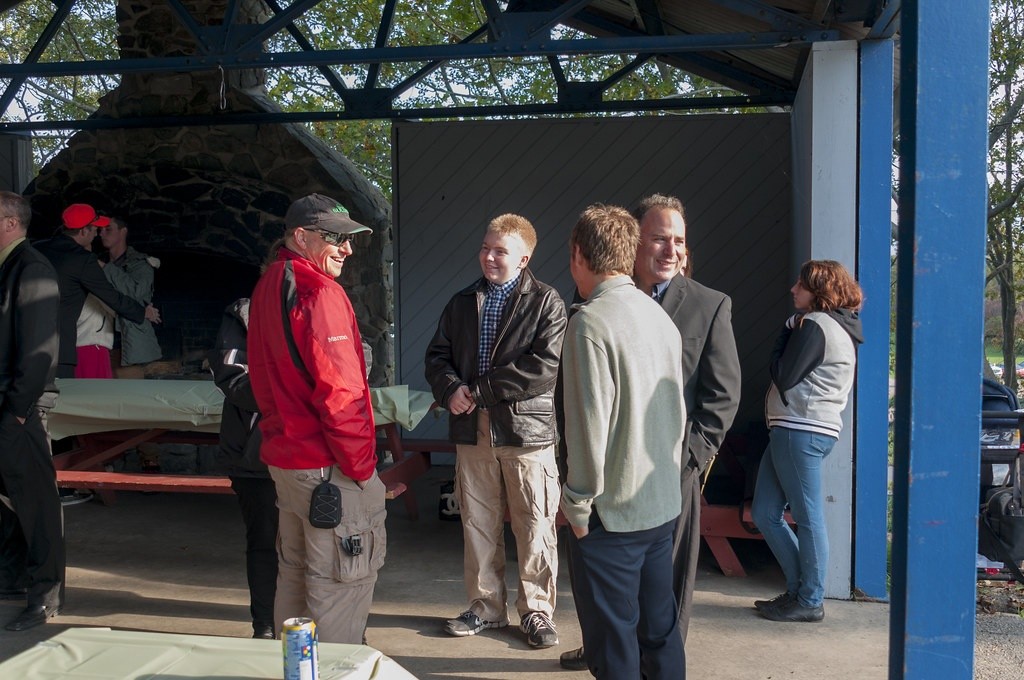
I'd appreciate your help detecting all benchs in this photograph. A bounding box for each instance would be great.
[741,500,796,527]
[49,431,458,500]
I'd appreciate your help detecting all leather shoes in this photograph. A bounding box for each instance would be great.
[560,647,591,670]
[760,602,825,624]
[0,587,30,601]
[6,605,64,631]
[753,591,796,611]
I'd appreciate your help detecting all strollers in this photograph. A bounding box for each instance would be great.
[977,375,1023,582]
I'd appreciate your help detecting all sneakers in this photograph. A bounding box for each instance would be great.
[445,611,511,636]
[517,614,559,649]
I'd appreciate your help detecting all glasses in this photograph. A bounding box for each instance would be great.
[290,228,355,245]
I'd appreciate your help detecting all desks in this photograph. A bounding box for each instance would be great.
[48,379,437,543]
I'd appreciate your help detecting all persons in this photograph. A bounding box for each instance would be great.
[0,193,65,632]
[247,192,388,643]
[49,204,161,506]
[752,260,865,622]
[556,205,687,680]
[425,214,567,648]
[210,222,281,639]
[560,197,741,670]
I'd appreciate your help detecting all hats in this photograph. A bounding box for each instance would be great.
[62,204,110,228]
[285,193,373,237]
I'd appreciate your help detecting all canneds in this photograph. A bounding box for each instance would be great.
[281,617,320,680]
